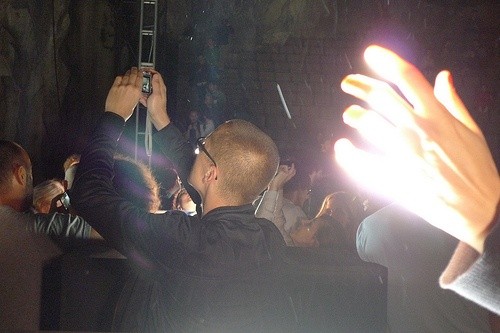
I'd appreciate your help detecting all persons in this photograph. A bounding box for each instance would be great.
[356,198,493,332]
[332,38,499,319]
[0,66,384,333]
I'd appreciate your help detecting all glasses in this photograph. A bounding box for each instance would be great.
[194,134,219,181]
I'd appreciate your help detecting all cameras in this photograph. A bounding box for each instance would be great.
[141,72,153,96]
[60,192,73,212]
